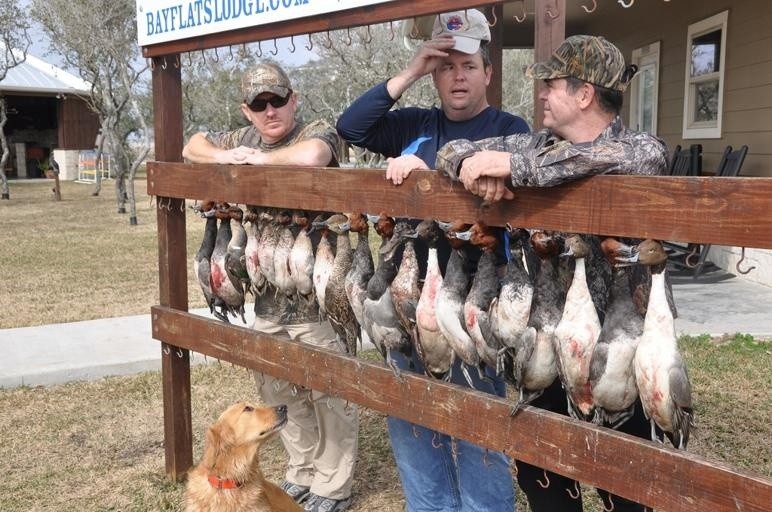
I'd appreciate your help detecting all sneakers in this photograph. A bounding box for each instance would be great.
[306,493,352,510]
[279,483,311,503]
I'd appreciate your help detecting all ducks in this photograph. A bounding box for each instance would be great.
[398,219,456,386]
[303,213,335,327]
[587,238,644,432]
[218,206,254,297]
[258,207,280,292]
[550,233,602,417]
[286,212,315,309]
[200,200,247,325]
[447,221,508,372]
[430,216,495,395]
[188,199,230,325]
[378,220,422,361]
[242,207,268,297]
[486,219,536,378]
[360,210,418,387]
[611,237,696,452]
[509,232,564,419]
[310,213,356,356]
[337,210,375,330]
[270,210,299,305]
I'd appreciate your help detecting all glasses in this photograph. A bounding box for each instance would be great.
[246,91,293,112]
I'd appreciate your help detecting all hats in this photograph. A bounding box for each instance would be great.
[432,9,491,54]
[523,36,628,91]
[242,60,292,105]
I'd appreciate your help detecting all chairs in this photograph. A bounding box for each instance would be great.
[662,145,748,285]
[667,144,694,261]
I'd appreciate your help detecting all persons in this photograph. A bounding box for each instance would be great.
[337,7,532,511]
[433,36,666,512]
[179,58,358,512]
